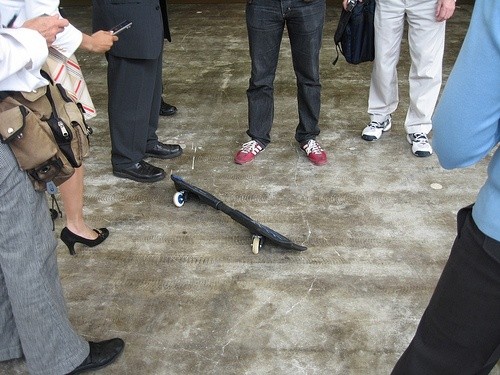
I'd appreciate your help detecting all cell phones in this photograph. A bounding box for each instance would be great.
[107,19,132,36]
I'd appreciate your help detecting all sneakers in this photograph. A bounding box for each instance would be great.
[300,139,327,164]
[235,141,265,164]
[65,338,125,375]
[362,114,392,142]
[406,132,433,157]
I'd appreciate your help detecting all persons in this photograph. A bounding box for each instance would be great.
[344,0,456,157]
[93,0,182,183]
[0,0,119,254]
[235,0,328,165]
[0,14,124,375]
[391,0,500,375]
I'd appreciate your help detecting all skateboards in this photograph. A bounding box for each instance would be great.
[170,173,308,254]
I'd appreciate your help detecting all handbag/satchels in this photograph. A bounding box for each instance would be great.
[333,0,375,65]
[0,69,93,193]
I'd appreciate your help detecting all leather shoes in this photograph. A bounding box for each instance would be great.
[113,160,167,183]
[158,102,177,116]
[144,142,182,159]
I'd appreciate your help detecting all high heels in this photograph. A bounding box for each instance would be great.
[50,209,60,230]
[60,227,109,255]
